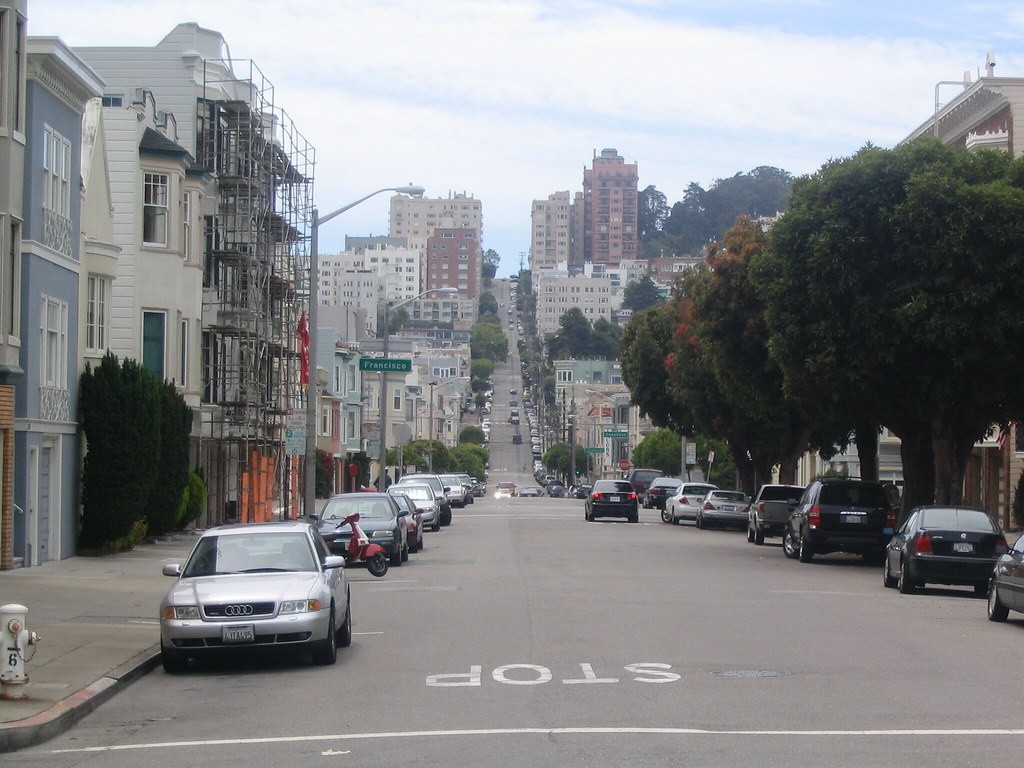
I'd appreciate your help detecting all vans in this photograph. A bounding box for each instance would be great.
[627,468,665,504]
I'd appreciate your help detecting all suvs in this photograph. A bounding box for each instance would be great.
[396,471,453,527]
[782,475,897,567]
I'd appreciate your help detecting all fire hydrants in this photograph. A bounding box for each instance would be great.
[0,603,41,699]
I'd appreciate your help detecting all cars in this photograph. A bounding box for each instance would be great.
[883,504,1010,599]
[584,479,640,524]
[495,481,517,498]
[695,488,751,532]
[661,482,722,525]
[479,272,594,501]
[385,482,443,532]
[377,491,425,554]
[643,477,684,510]
[310,491,410,567]
[158,520,353,675]
[986,532,1024,623]
[517,486,540,497]
[435,472,486,509]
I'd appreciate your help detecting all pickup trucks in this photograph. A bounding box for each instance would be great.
[746,483,806,545]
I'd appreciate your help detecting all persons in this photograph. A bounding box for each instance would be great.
[374,469,392,493]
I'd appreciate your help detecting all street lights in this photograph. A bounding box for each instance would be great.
[584,389,618,480]
[303,183,426,520]
[377,287,459,492]
[428,376,471,474]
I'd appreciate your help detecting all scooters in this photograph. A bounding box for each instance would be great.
[328,511,388,578]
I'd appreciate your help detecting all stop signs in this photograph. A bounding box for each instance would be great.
[619,459,630,471]
